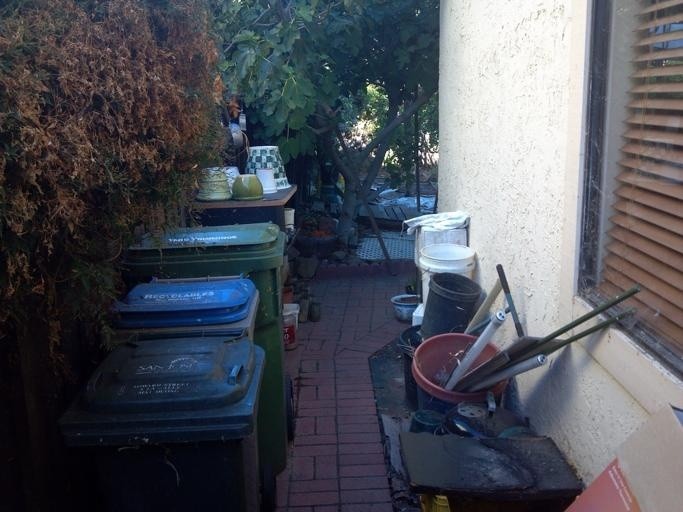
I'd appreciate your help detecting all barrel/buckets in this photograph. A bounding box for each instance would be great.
[255,167,278,194]
[283,304,300,349]
[245,145,291,189]
[284,207,295,231]
[400,217,511,416]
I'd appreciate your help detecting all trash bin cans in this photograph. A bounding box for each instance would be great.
[54,328,267,512]
[120,221,295,476]
[110,273,260,340]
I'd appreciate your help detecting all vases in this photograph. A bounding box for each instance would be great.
[390,294,421,323]
[196,145,293,201]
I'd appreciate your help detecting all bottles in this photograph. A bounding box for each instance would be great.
[239,113,246,131]
